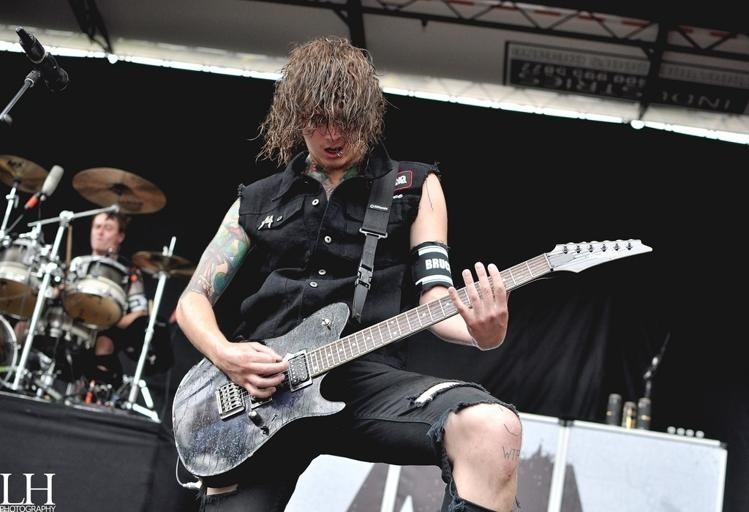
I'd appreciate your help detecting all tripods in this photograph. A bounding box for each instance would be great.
[0,220,170,426]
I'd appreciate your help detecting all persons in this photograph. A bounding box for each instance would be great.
[2,209,152,404]
[162,34,524,511]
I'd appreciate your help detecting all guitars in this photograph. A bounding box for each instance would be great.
[172,237,652,487]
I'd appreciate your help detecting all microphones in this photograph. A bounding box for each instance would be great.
[15,27,71,95]
[39,165,64,204]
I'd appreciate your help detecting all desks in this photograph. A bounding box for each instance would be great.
[284,411,729,512]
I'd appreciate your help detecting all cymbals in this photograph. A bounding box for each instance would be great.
[0,155,47,193]
[134,252,196,275]
[73,168,166,214]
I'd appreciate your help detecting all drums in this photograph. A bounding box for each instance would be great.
[63,256,132,328]
[14,299,96,362]
[0,237,63,320]
